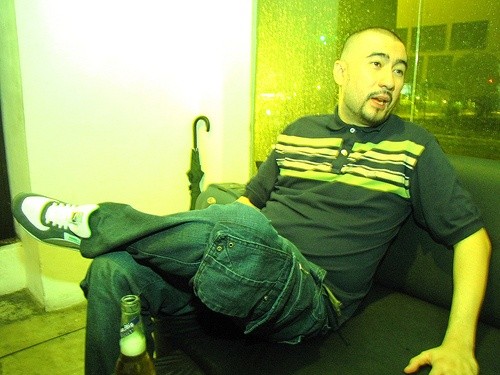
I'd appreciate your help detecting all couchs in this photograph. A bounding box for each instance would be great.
[156,149,500,375]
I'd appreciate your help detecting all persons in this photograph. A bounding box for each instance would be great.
[11,26,491,375]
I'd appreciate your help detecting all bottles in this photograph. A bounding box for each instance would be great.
[112,295,156,375]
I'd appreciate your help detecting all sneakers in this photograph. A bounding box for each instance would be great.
[11,193,98,250]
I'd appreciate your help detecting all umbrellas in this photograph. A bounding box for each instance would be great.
[187,115,212,211]
